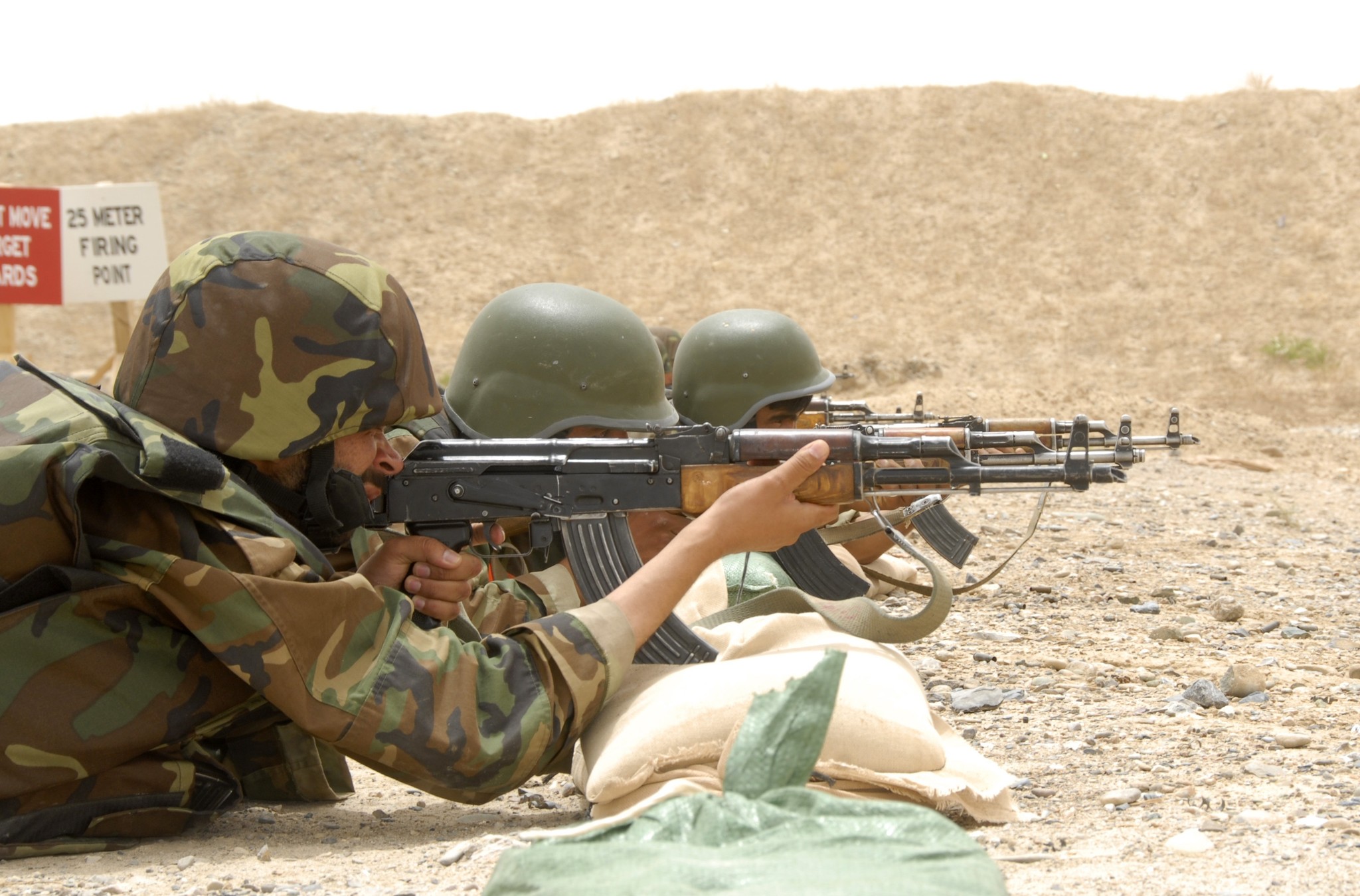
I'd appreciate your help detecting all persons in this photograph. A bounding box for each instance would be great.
[1,225,841,859]
[647,327,683,387]
[667,307,1028,565]
[353,281,955,644]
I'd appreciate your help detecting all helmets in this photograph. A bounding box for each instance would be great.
[672,309,837,433]
[444,283,679,439]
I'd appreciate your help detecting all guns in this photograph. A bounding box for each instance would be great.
[360,394,1199,665]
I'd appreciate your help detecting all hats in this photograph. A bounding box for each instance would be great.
[113,230,443,461]
